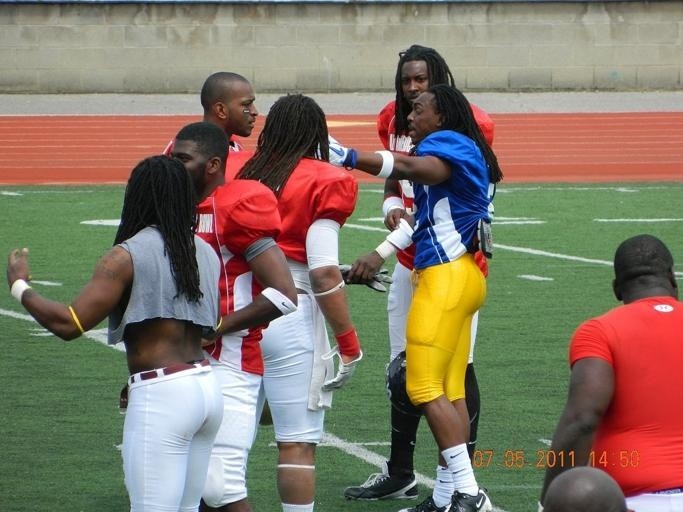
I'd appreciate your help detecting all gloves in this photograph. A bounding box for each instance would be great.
[321,346,363,391]
[340,263,392,292]
[317,132,357,171]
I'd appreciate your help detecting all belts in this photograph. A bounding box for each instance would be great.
[128,359,210,385]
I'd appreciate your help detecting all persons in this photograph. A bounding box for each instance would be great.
[537,235,683,512]
[541,466,627,512]
[8,154,225,512]
[234,94,364,512]
[173,122,298,510]
[343,44,493,502]
[162,72,259,181]
[328,85,503,512]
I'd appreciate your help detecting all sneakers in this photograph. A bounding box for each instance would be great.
[449,490,492,512]
[345,460,419,500]
[398,497,449,512]
